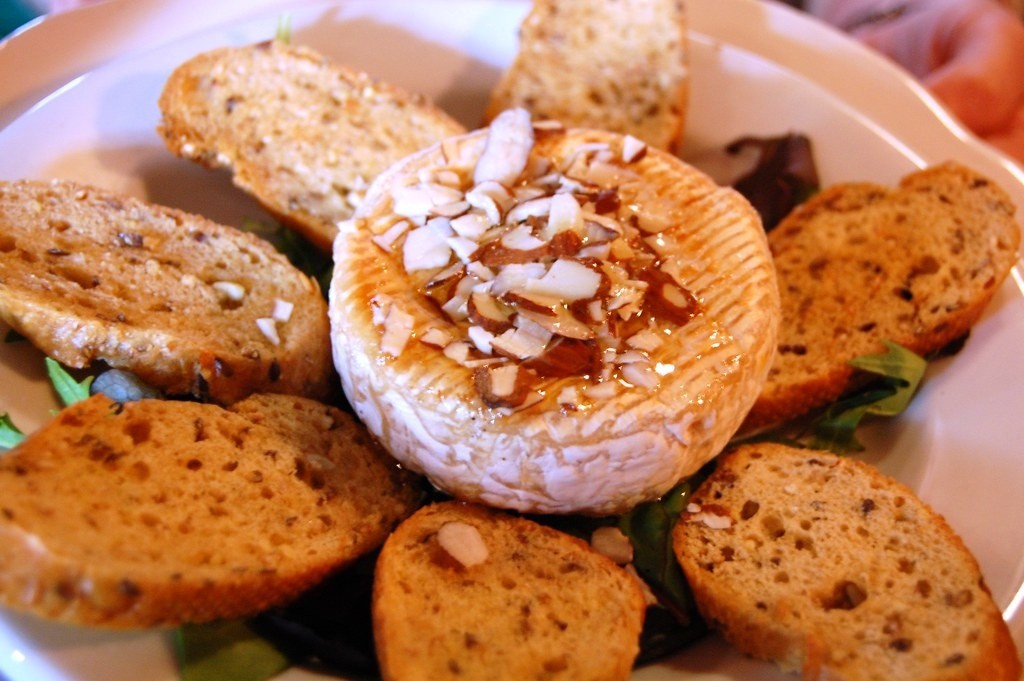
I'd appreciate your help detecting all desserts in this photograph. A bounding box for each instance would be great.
[322,103,783,512]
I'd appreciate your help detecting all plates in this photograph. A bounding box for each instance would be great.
[0,0,1024,680]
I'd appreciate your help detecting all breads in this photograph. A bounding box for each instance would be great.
[0,0,1024,681]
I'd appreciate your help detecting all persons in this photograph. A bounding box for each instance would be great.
[800,1,1024,170]
